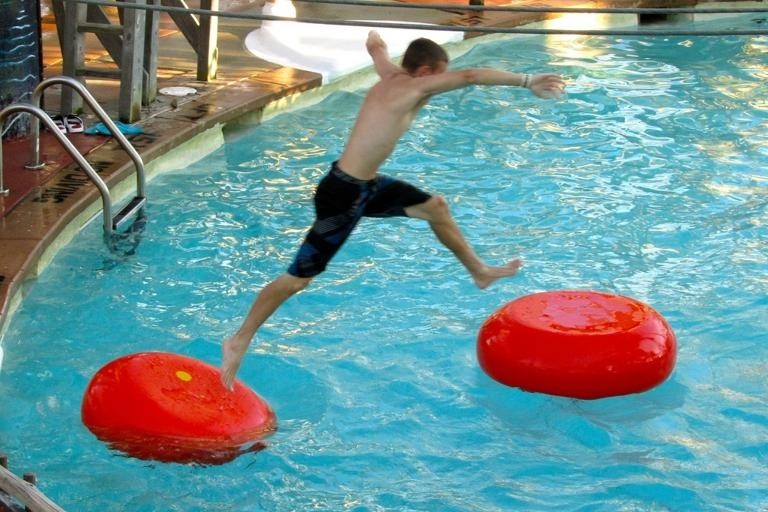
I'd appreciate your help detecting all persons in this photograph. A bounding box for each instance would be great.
[219,30,567,393]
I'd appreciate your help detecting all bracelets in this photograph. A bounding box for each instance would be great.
[521,74,532,88]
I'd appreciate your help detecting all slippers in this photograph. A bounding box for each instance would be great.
[96,120,144,136]
[63,112,84,133]
[50,113,67,135]
[84,123,103,134]
